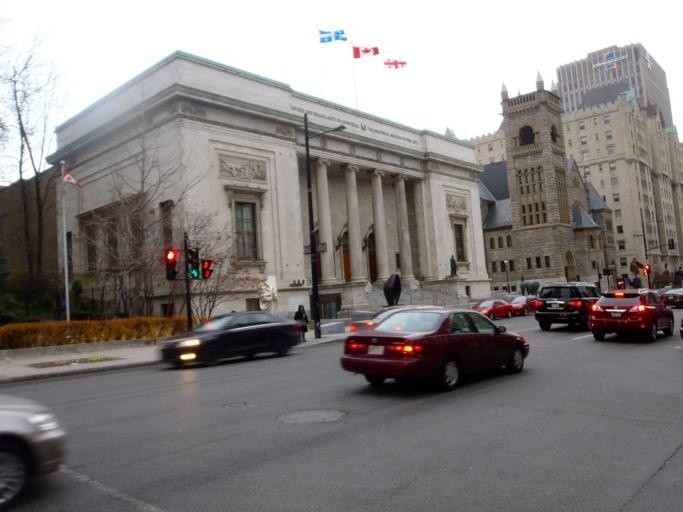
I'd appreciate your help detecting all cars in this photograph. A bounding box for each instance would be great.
[661,288,683,307]
[162,310,305,369]
[472,294,537,320]
[341,303,530,392]
[591,288,674,342]
[0,394,68,512]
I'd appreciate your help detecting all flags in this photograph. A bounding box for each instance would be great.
[383,58,406,68]
[319,30,346,41]
[352,46,379,59]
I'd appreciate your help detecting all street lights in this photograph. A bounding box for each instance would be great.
[306,125,346,339]
[642,220,663,289]
[504,260,511,293]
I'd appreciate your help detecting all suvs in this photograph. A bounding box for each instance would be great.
[534,282,602,331]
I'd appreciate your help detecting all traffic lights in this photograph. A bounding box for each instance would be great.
[617,278,624,286]
[201,258,213,279]
[644,263,650,277]
[165,247,178,280]
[186,247,200,279]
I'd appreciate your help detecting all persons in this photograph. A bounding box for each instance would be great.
[630,257,643,278]
[294,305,308,342]
[449,254,456,277]
[51,293,66,320]
[650,266,683,288]
